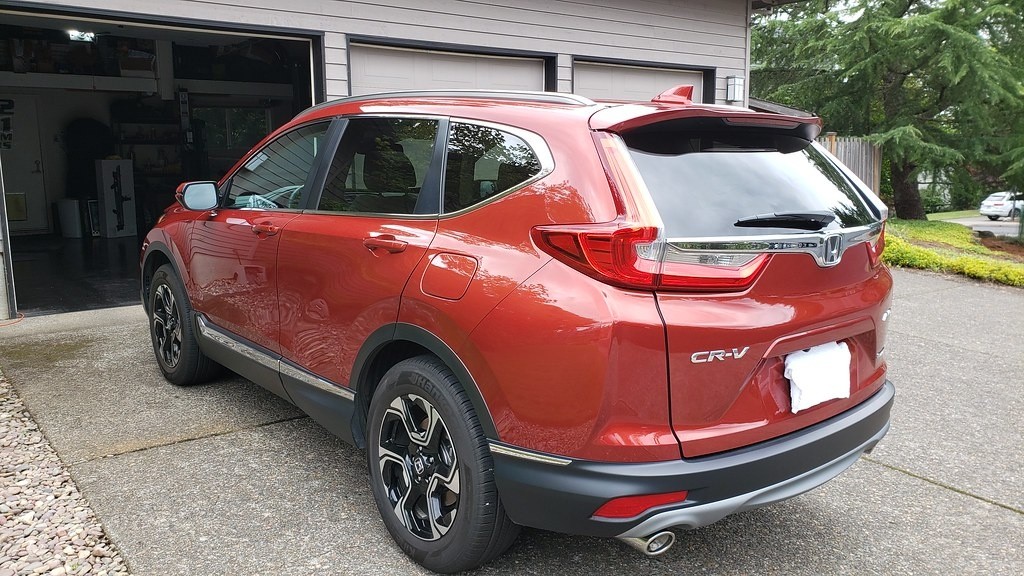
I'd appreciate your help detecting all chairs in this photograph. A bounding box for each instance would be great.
[347,149,419,213]
[444,151,527,211]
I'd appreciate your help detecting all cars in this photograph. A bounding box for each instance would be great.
[980,191,1024,221]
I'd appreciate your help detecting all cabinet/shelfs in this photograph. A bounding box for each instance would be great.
[110,116,184,194]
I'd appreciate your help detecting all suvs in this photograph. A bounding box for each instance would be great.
[137,85,898,576]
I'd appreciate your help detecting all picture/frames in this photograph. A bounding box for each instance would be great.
[87,199,101,237]
